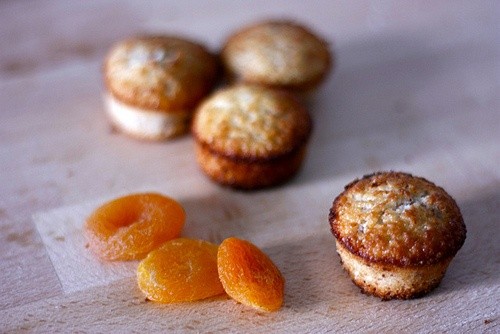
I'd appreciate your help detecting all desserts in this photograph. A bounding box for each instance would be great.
[101,35,216,142]
[217,20,333,93]
[329,171,468,301]
[189,84,314,192]
